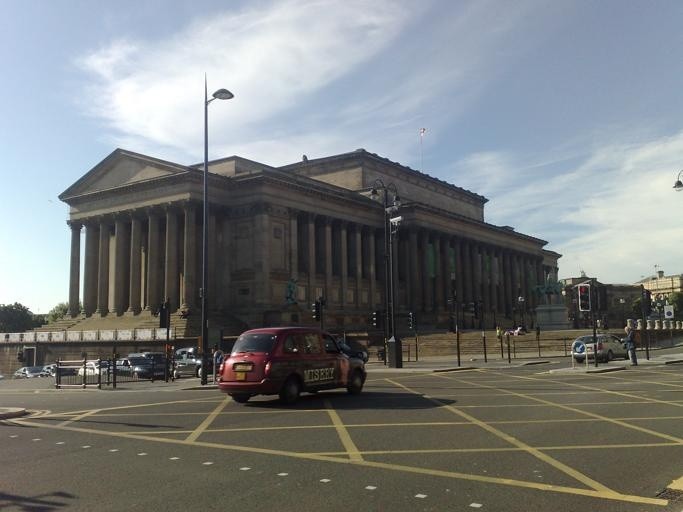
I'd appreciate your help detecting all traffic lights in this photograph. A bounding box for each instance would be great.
[312,300,320,322]
[407,312,414,331]
[371,310,380,329]
[578,284,591,312]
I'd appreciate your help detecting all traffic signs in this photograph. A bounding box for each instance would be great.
[664,306,674,318]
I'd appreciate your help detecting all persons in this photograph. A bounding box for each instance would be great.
[213,346,227,383]
[620,326,638,366]
[495,320,541,344]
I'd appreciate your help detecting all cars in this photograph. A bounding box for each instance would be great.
[217,327,367,402]
[571,334,629,363]
[12,346,231,380]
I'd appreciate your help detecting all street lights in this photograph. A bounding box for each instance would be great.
[202,72,235,385]
[368,179,403,368]
